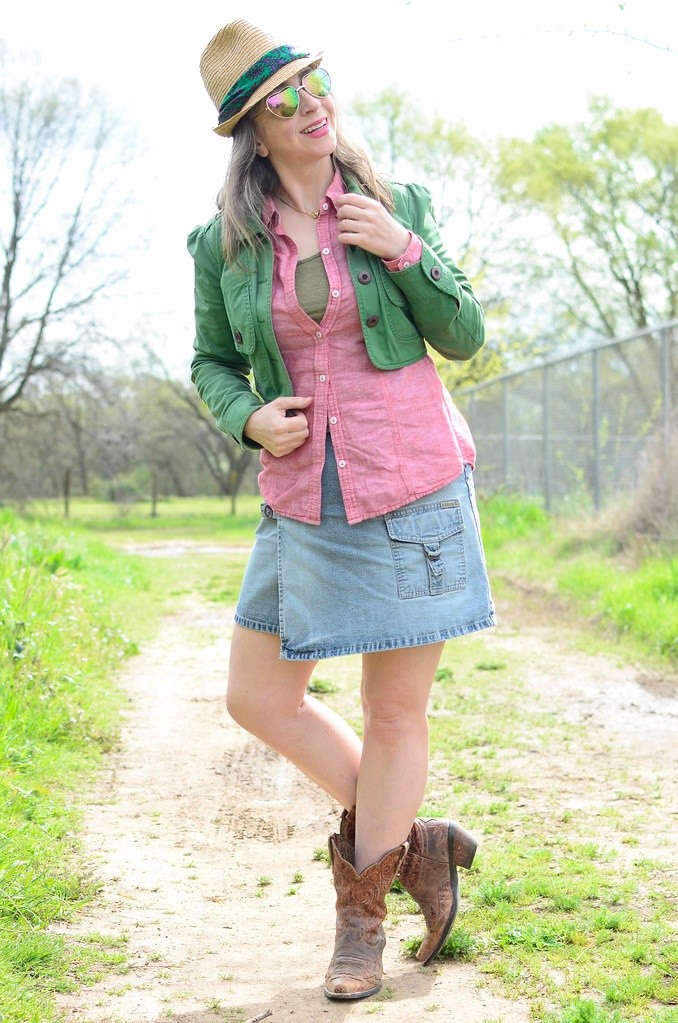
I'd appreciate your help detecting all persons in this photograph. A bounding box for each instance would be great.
[186,21,499,1000]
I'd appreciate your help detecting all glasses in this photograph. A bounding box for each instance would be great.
[251,66,331,125]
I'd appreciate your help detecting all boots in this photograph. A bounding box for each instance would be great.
[323,807,408,999]
[339,799,477,965]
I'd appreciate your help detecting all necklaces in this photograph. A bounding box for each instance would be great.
[274,191,321,219]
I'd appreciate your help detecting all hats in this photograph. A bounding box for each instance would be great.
[199,21,322,136]
